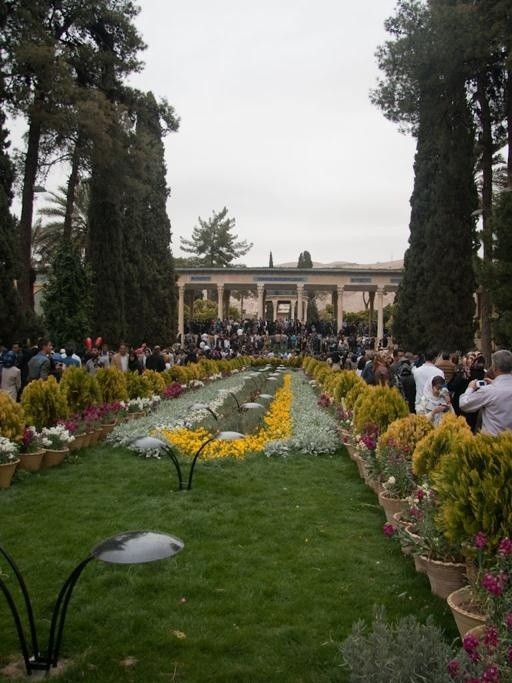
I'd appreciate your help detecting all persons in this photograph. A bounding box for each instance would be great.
[356,345,485,434]
[181,314,391,372]
[459,349,512,437]
[0,337,181,403]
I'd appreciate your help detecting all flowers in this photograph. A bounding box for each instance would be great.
[1,363,206,490]
